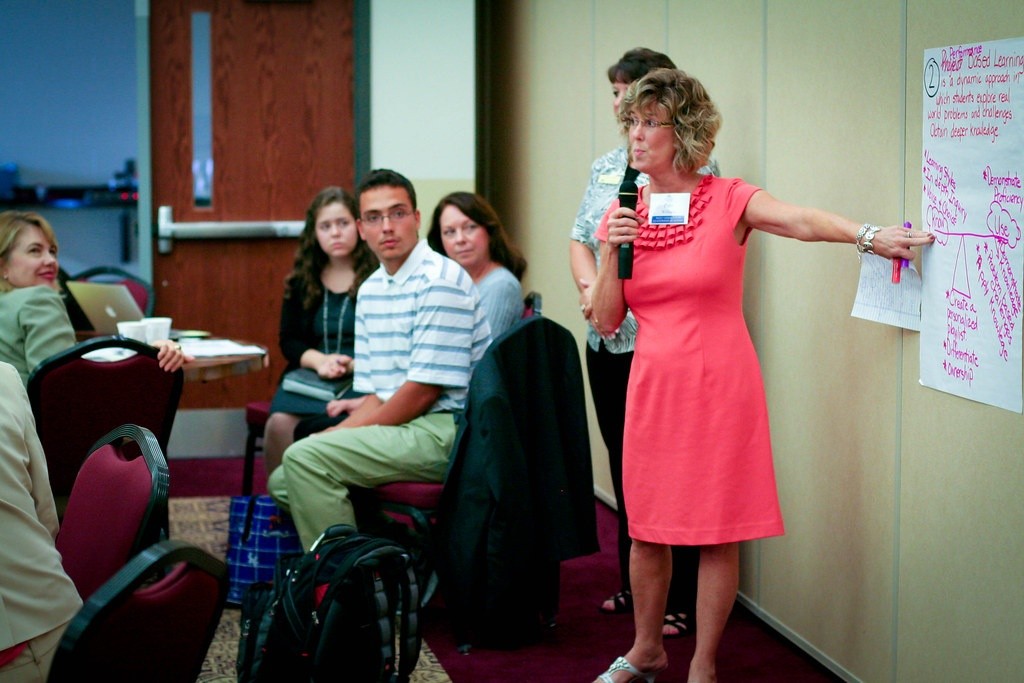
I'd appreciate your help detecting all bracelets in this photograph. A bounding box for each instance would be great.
[856,223,881,266]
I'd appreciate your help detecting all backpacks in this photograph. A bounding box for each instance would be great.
[237,524,422,683]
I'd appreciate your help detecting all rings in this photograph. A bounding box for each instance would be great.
[176,345,181,349]
[908,232,912,238]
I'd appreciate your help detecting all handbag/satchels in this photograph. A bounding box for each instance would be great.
[224,493,304,610]
[283,368,355,401]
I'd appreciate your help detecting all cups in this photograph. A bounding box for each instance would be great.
[142,316,174,342]
[117,321,148,345]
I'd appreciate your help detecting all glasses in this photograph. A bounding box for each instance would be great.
[622,118,675,131]
[360,208,413,226]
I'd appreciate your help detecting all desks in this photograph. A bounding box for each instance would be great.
[81,338,267,383]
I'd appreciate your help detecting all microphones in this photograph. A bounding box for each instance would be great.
[617,181,639,279]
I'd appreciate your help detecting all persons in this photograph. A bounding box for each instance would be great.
[267,169,493,557]
[0,210,194,390]
[589,69,936,683]
[569,49,721,636]
[427,192,528,341]
[263,186,380,477]
[0,361,83,683]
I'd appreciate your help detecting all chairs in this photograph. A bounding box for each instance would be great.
[239,293,598,653]
[45,540,233,683]
[27,335,185,526]
[71,266,154,318]
[55,424,169,604]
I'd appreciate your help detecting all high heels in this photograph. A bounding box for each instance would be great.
[597,656,668,683]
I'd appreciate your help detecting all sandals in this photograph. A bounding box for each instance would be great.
[600,590,634,614]
[661,613,688,638]
